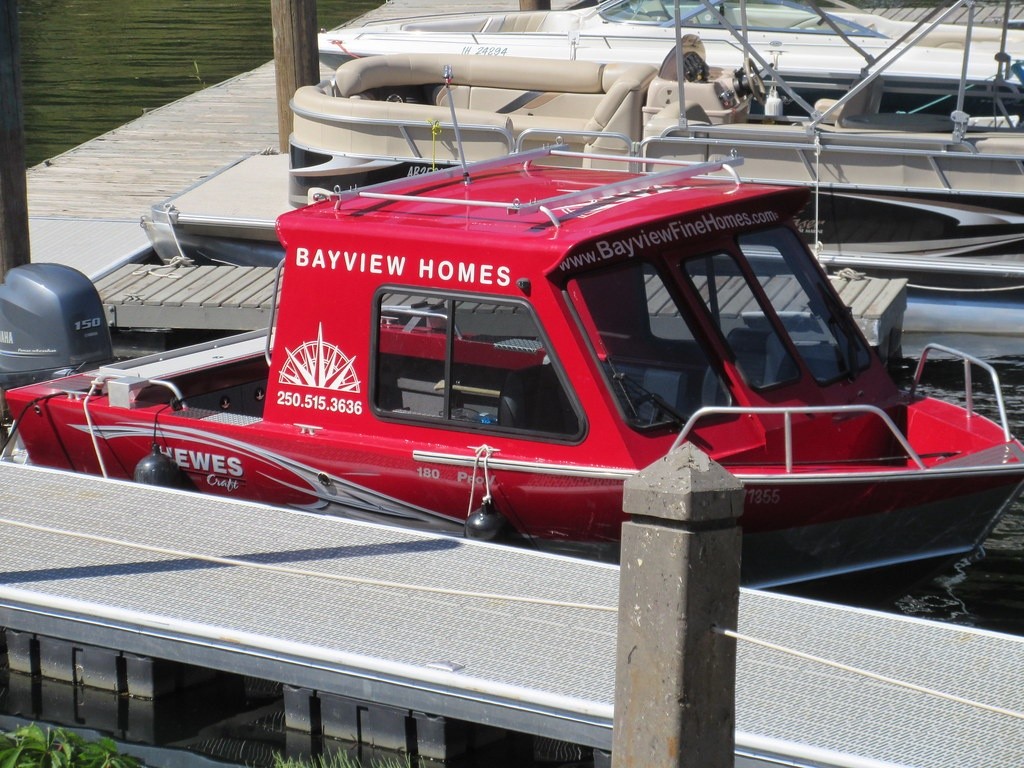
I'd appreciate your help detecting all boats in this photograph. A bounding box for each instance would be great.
[0,136,1024,591]
[140,0,1024,359]
